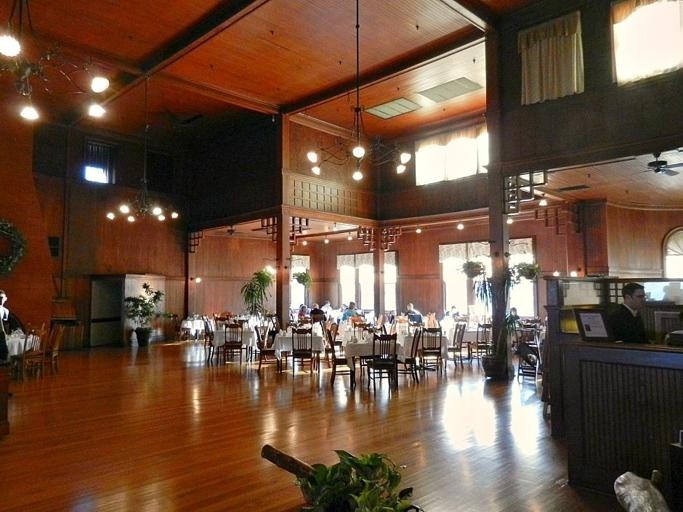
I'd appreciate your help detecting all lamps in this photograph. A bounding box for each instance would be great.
[0,0,112,128]
[305,1,413,186]
[99,75,180,224]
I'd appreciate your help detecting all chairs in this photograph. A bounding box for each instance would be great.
[177,308,547,396]
[0,318,65,385]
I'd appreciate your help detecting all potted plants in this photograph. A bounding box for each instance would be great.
[460,261,485,281]
[510,262,543,283]
[121,281,174,348]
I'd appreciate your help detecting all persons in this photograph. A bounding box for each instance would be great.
[405,303,423,323]
[0,290,13,399]
[603,282,653,345]
[298,300,362,323]
[506,307,520,325]
[445,305,462,324]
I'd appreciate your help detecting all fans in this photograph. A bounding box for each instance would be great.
[210,225,247,238]
[631,152,683,177]
